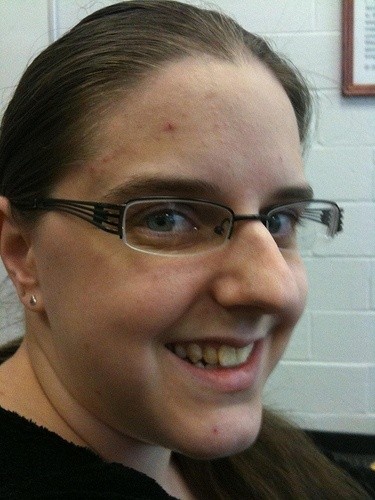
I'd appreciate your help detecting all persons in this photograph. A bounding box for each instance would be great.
[0,0,375,500]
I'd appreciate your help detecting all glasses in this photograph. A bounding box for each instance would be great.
[12,197,344,258]
